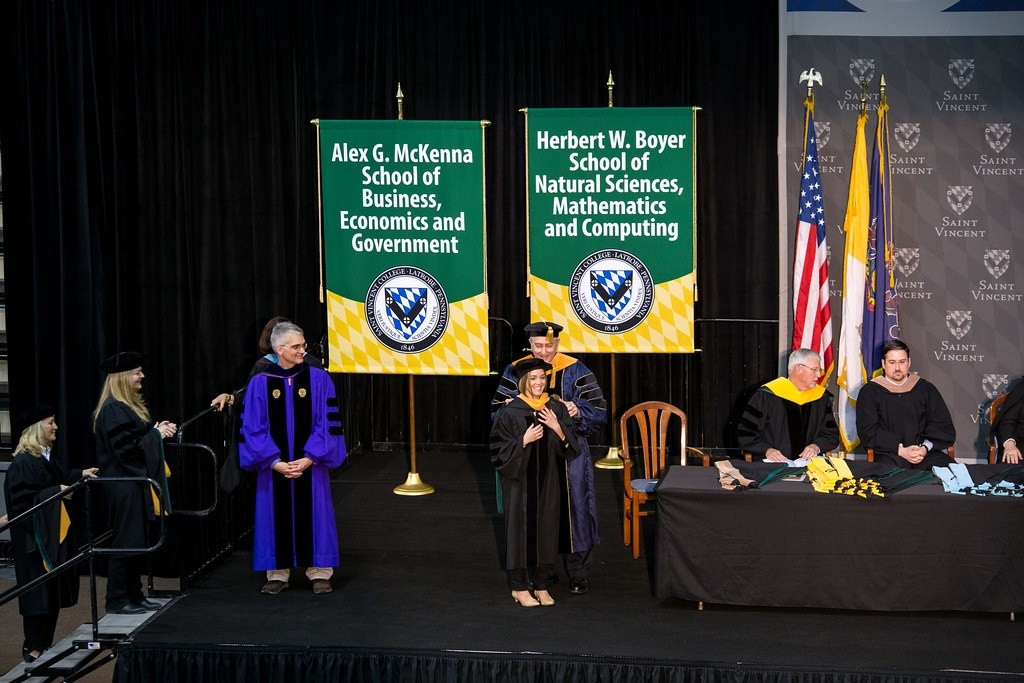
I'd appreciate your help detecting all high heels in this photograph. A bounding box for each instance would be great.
[511,590,540,607]
[534,589,556,605]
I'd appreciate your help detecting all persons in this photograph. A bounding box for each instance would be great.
[210,316,347,595]
[737,348,840,461]
[489,358,581,606]
[989,376,1024,464]
[856,340,956,464]
[4,405,99,662]
[492,322,606,594]
[93,352,177,614]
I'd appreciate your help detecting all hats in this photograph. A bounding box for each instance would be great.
[101,352,144,373]
[524,322,563,344]
[510,358,556,388]
[18,405,54,434]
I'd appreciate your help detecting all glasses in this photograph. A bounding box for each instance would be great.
[280,344,307,349]
[799,364,824,373]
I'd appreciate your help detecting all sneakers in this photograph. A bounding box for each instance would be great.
[313,579,332,594]
[261,580,289,594]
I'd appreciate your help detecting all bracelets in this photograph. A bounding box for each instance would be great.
[226,395,231,403]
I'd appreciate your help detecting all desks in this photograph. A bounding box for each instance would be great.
[653,465,1024,620]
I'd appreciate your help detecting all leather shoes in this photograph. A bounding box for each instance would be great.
[106,600,146,613]
[137,600,161,610]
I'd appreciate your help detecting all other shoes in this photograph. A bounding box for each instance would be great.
[571,578,588,594]
[543,573,560,586]
[23,653,42,662]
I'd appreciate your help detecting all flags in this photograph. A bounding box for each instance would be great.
[794,108,900,453]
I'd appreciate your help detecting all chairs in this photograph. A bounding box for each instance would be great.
[985,392,1008,464]
[867,445,955,462]
[620,400,710,558]
[743,449,833,462]
